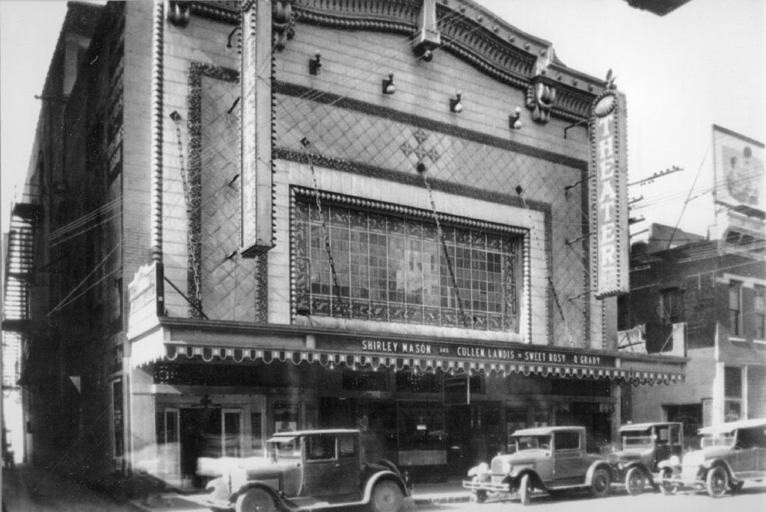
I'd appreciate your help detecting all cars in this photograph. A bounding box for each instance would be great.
[196,429,410,511]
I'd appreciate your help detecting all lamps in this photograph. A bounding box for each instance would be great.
[309,48,322,74]
[507,106,523,130]
[382,73,397,94]
[449,94,464,113]
